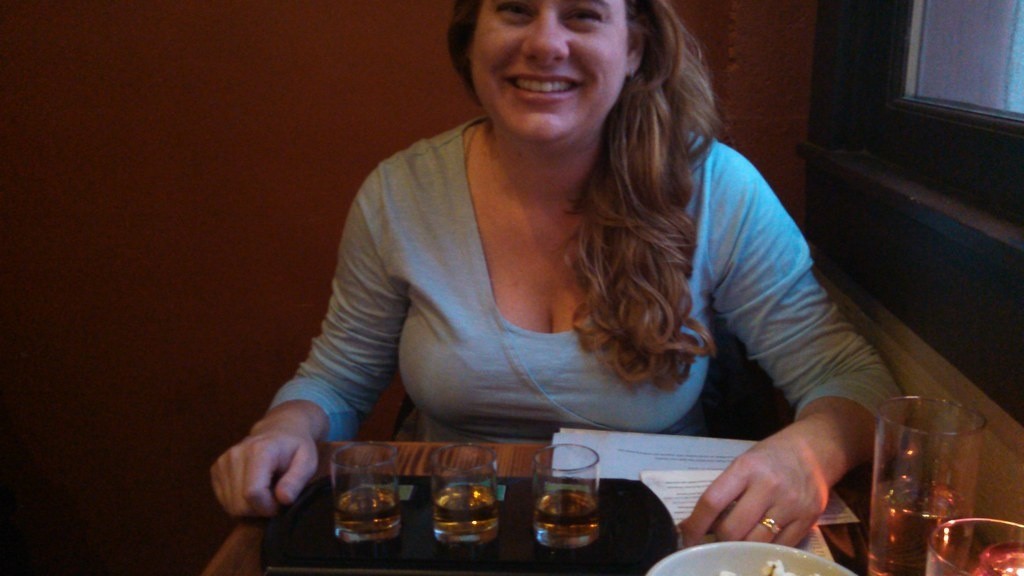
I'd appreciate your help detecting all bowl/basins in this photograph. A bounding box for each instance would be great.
[646,541,858,576]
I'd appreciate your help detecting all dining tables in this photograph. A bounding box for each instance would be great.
[202,431,1024,576]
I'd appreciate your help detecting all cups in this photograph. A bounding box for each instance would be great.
[926,518,1024,576]
[867,394,987,576]
[428,444,498,549]
[330,444,401,543]
[534,444,599,552]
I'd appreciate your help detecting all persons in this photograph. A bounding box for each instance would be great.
[210,0,908,553]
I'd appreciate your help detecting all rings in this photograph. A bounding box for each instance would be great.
[760,514,780,533]
